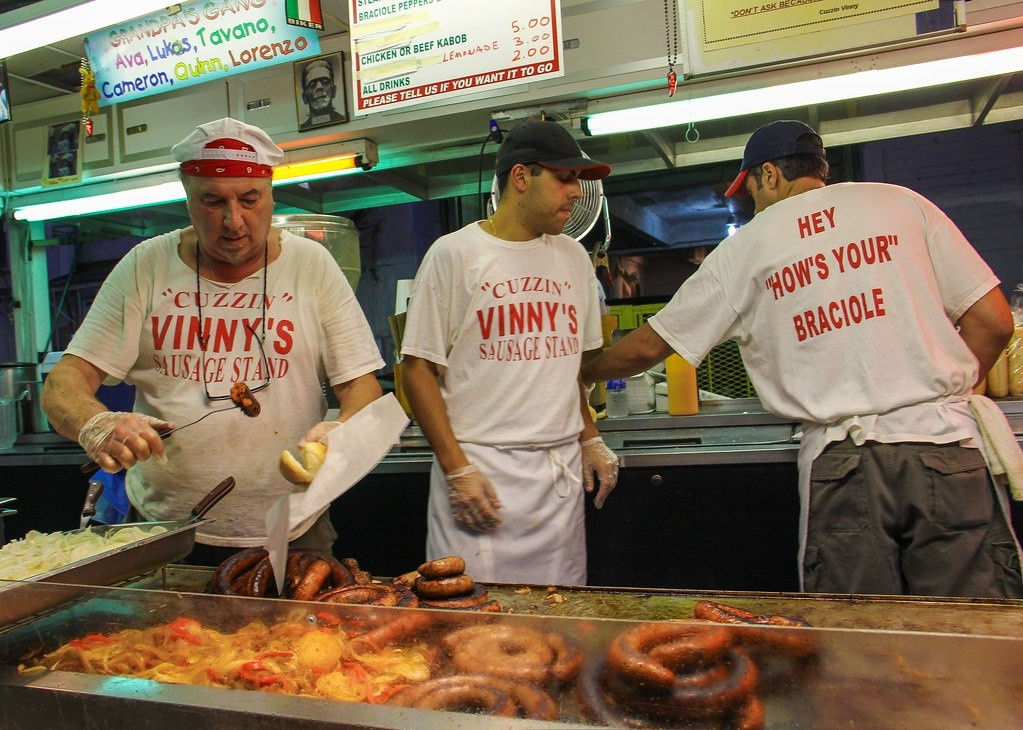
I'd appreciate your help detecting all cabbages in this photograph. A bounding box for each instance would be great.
[0,525,169,586]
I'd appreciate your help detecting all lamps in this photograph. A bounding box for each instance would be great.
[572,25,1023,137]
[7,140,379,223]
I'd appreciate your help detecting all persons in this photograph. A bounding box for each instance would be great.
[580,119,1023,599]
[399,119,620,586]
[40,117,386,567]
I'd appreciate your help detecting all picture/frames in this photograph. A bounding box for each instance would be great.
[293,50,348,132]
[41,118,83,188]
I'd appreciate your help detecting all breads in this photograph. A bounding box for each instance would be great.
[973,327,1023,398]
[280,442,327,485]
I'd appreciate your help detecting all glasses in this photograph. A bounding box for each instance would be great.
[204,325,272,400]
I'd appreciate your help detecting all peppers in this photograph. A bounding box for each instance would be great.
[67,611,414,701]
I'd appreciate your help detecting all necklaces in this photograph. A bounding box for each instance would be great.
[488,214,496,236]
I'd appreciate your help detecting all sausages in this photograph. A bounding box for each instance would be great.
[230,382,261,417]
[212,546,824,729]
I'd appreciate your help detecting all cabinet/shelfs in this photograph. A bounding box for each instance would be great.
[0,449,800,597]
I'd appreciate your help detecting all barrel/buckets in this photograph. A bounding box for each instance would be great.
[0,362,44,448]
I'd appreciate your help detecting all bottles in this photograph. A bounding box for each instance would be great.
[665,352,699,415]
[605,378,630,419]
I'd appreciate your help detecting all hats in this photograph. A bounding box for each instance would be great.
[724,120,827,197]
[495,117,611,181]
[172,116,285,166]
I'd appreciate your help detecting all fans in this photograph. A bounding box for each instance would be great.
[492,151,611,255]
